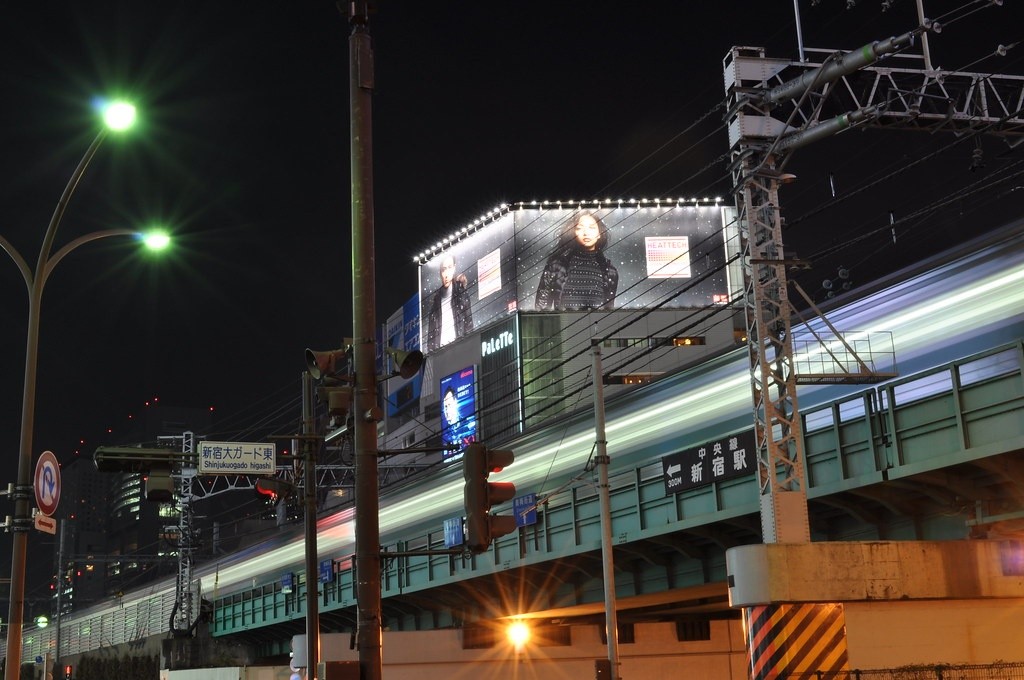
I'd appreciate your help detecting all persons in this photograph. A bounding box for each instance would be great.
[534,208,619,310]
[444,385,476,455]
[426,253,473,351]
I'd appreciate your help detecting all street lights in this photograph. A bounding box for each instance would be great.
[0,95,177,680]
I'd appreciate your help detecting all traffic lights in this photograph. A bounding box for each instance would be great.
[254,476,282,506]
[461,442,517,555]
